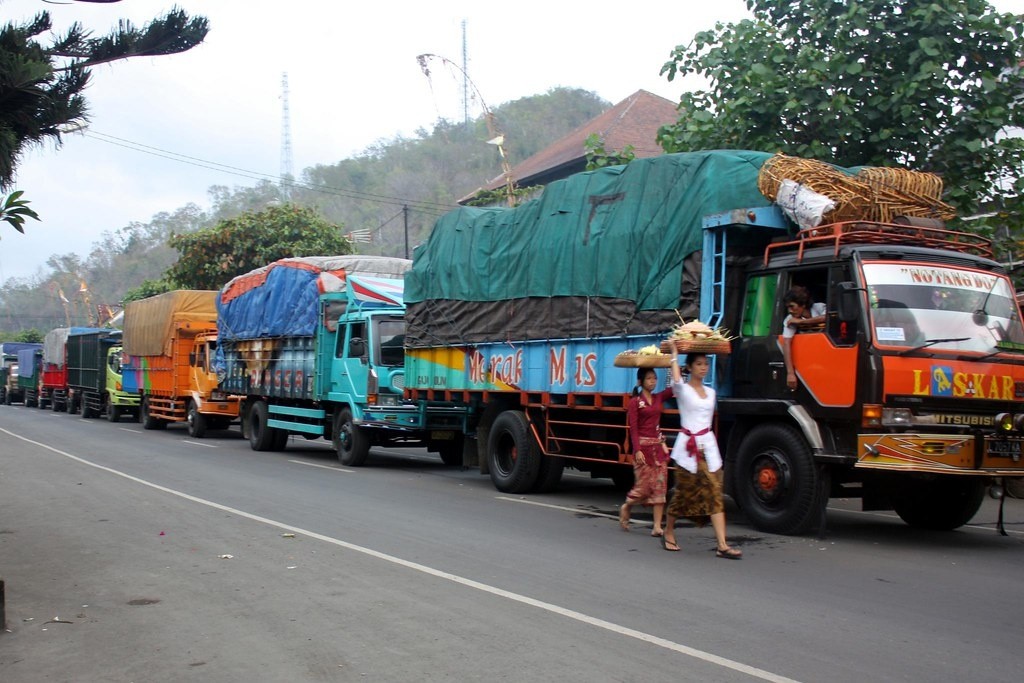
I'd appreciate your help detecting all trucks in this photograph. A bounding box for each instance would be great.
[122,289,248,439]
[402,151,1023,532]
[45,328,80,414]
[0,353,24,405]
[18,349,52,409]
[67,329,142,424]
[214,256,473,468]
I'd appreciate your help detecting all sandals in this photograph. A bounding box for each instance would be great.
[716,548,742,559]
[651,529,662,537]
[620,517,630,525]
[663,534,680,551]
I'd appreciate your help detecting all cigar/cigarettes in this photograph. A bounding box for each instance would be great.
[791,388,795,391]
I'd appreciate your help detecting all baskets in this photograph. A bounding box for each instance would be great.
[615,356,672,368]
[660,341,731,354]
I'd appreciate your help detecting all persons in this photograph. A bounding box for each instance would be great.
[781,286,827,389]
[660,339,743,559]
[618,366,674,537]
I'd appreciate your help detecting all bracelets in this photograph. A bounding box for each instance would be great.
[669,359,678,364]
